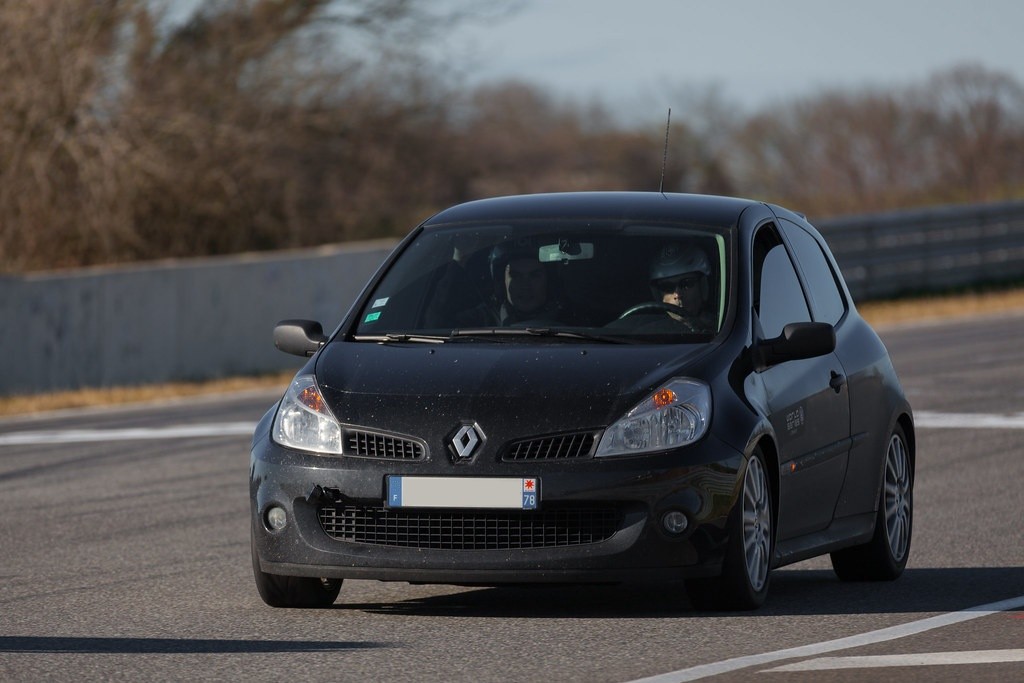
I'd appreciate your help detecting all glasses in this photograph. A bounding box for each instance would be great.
[654,273,705,294]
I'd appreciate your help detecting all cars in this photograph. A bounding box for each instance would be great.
[248,192,916,611]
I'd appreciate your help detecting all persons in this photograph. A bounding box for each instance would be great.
[423,240,567,329]
[643,244,718,333]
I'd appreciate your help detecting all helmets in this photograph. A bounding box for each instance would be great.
[649,241,712,281]
[489,229,567,282]
